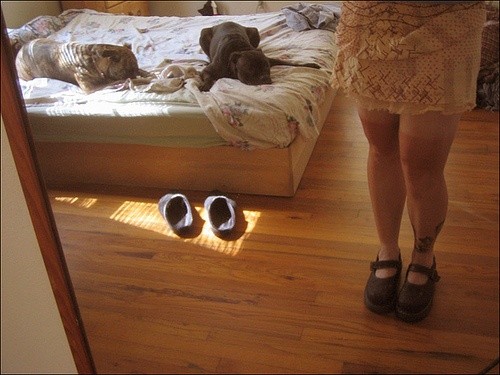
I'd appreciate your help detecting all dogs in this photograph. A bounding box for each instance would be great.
[198,21,322,92]
[14,37,157,96]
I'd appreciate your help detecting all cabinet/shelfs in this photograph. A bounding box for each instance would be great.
[59,1,153,17]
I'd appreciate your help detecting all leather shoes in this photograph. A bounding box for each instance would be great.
[364,249,401,314]
[396,254,441,324]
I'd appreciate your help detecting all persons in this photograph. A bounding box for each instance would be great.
[329,2,486,323]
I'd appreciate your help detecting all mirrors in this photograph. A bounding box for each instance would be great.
[0,0,499,375]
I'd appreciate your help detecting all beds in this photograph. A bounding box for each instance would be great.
[10,7,343,196]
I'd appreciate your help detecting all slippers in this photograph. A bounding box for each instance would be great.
[158,193,193,237]
[204,190,239,237]
